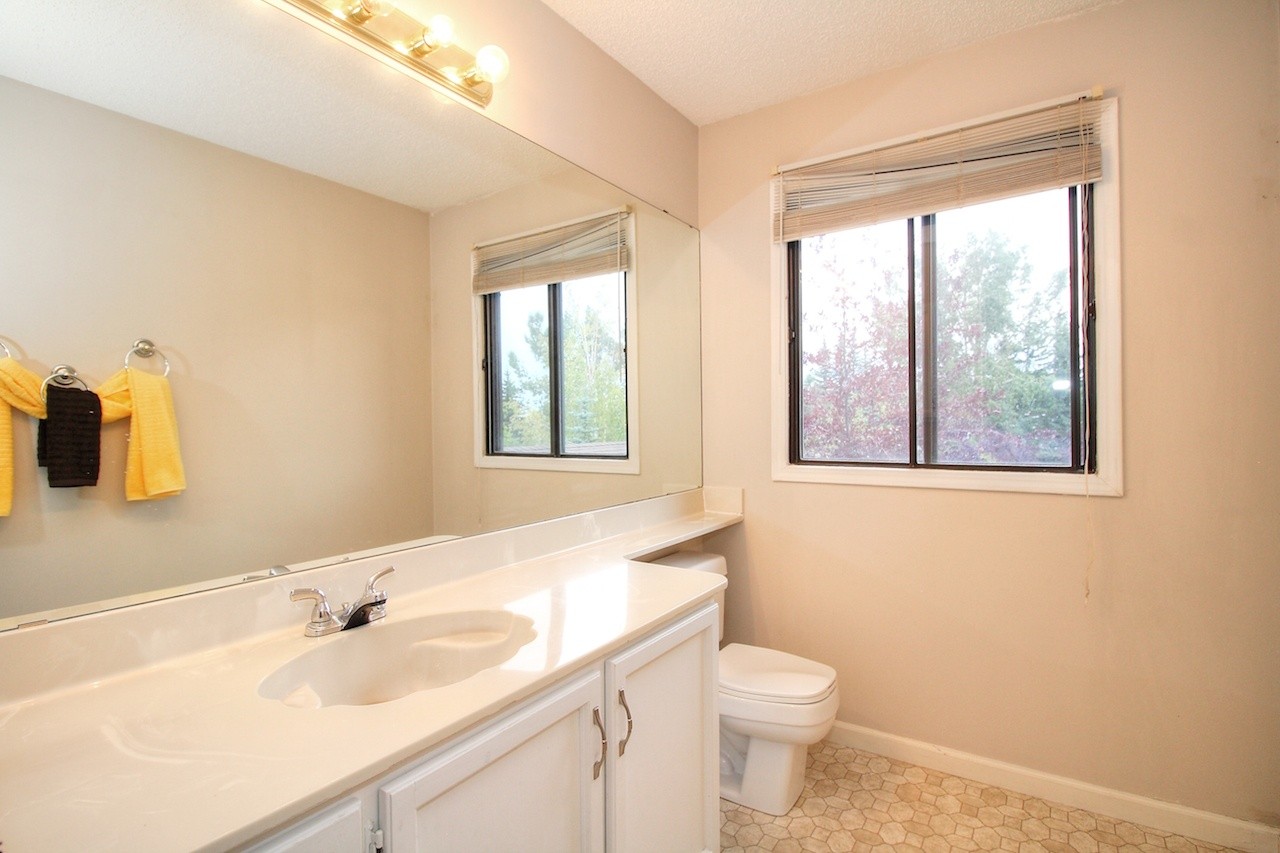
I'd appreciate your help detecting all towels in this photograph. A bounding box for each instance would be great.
[0,356,187,517]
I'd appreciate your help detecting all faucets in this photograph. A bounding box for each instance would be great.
[340,591,389,631]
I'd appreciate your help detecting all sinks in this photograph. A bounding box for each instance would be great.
[256,608,538,707]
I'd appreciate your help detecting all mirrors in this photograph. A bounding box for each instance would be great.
[0,1,704,628]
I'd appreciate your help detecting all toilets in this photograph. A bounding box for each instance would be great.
[647,551,842,817]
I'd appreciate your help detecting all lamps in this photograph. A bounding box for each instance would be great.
[290,0,509,107]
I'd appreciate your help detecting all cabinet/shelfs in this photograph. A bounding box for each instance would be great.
[380,604,722,853]
